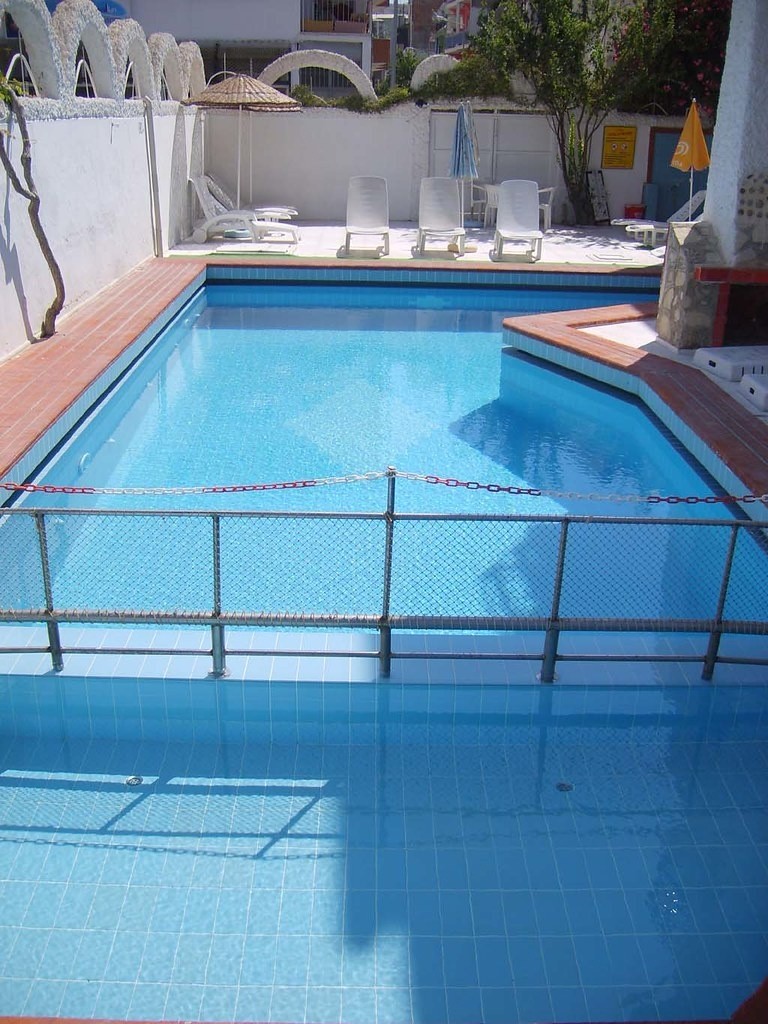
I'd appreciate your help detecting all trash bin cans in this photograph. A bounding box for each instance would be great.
[624,204,646,219]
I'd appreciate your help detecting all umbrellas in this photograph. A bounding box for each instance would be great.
[451,98,482,227]
[180,71,305,210]
[671,96,709,223]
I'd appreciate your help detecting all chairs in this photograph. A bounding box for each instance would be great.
[189,173,299,243]
[417,178,466,256]
[493,180,544,259]
[539,188,555,230]
[346,175,389,255]
[484,183,500,227]
[625,213,703,246]
[611,189,707,241]
[474,185,485,227]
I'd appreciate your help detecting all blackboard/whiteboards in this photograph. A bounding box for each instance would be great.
[586,170,611,222]
